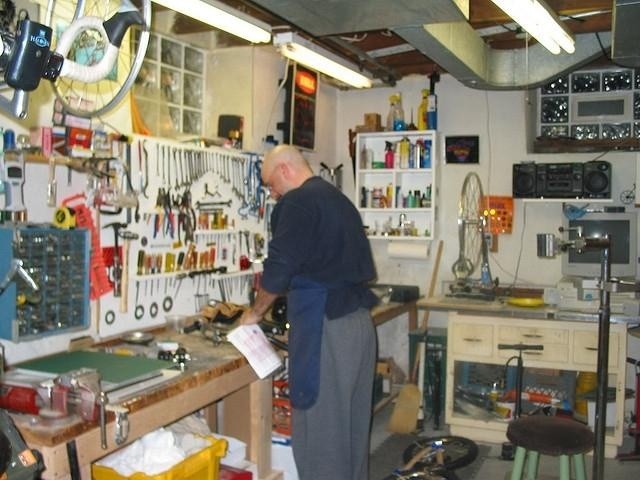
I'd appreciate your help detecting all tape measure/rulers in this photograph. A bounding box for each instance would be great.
[53,206,76,231]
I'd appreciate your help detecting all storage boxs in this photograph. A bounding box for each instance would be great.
[90,421,231,480]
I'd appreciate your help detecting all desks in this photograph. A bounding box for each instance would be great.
[197,288,423,480]
[2,318,287,480]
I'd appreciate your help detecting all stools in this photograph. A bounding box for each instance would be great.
[407,324,448,424]
[503,413,598,479]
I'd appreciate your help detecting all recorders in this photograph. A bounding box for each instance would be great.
[513,160,612,198]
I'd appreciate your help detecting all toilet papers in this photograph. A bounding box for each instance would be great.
[386,243,431,261]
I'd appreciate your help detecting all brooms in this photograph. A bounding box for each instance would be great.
[383,240,444,435]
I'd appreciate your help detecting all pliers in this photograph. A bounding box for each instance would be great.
[153,187,197,246]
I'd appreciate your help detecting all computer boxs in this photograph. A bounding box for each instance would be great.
[560,275,640,313]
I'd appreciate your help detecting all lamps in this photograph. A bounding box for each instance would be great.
[489,0,576,57]
[152,0,276,49]
[271,26,371,90]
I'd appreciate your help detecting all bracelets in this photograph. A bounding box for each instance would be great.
[249,306,262,317]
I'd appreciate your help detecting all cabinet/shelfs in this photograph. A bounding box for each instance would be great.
[1,221,95,343]
[83,24,208,148]
[355,129,441,243]
[416,293,635,460]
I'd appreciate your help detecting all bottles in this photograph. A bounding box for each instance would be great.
[361,183,393,209]
[364,145,372,169]
[382,213,415,236]
[387,93,405,131]
[395,136,431,169]
[396,183,431,208]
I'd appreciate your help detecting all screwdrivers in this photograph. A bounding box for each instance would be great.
[136,244,215,303]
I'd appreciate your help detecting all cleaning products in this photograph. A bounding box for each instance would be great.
[416,88,429,129]
[387,96,400,129]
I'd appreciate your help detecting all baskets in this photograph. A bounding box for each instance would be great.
[462,362,517,392]
[523,368,575,410]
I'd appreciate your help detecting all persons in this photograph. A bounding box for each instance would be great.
[235,142,380,480]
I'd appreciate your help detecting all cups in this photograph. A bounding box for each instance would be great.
[166,316,186,334]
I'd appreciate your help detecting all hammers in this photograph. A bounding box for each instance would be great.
[101,223,138,313]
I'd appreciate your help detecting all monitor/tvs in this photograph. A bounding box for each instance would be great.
[562,212,638,278]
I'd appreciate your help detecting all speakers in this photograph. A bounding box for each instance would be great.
[611,1,640,67]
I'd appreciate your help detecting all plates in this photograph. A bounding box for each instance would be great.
[120,332,154,344]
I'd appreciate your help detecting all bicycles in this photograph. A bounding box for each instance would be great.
[380,435,477,479]
